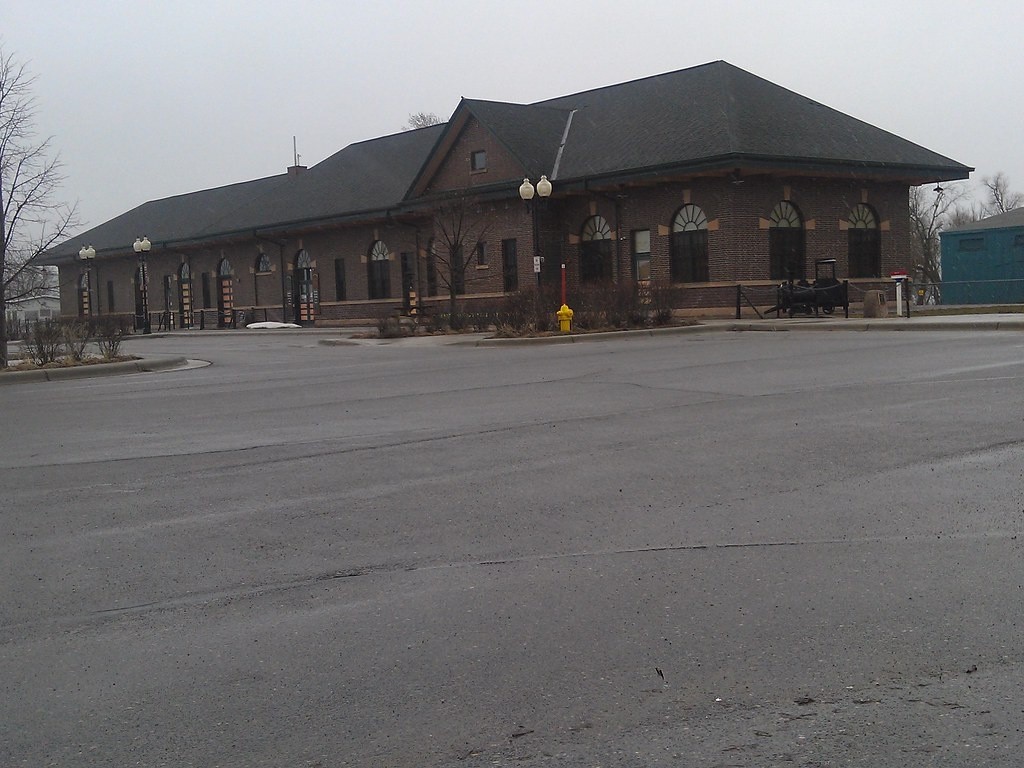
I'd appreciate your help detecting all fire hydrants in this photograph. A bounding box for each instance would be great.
[557,303,573,331]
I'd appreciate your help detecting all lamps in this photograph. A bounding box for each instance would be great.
[918,289,924,296]
[933,182,943,192]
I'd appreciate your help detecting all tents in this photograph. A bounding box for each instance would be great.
[939,207,1024,304]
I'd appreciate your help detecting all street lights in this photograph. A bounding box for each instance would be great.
[78,243,95,315]
[519,171,553,290]
[132,234,152,334]
